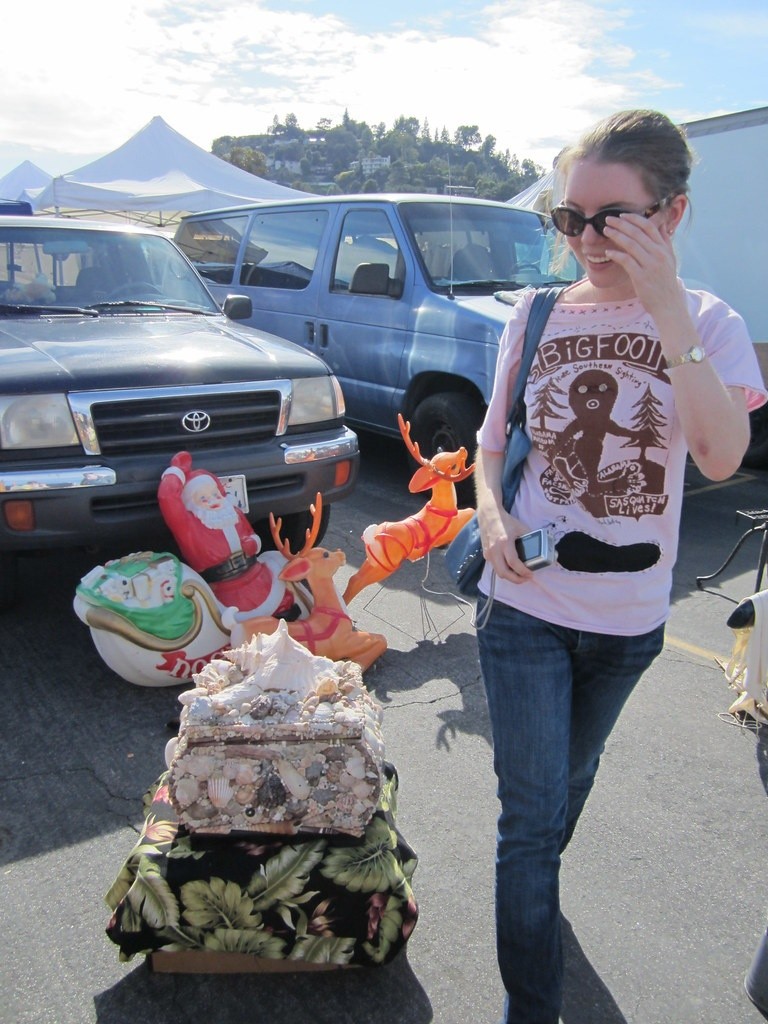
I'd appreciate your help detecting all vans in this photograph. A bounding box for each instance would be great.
[172,194,579,504]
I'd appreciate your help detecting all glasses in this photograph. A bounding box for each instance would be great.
[550,192,678,238]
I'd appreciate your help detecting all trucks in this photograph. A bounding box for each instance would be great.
[549,105,768,469]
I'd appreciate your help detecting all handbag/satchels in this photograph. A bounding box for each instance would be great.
[446,287,566,597]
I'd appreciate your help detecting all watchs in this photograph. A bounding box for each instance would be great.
[666,344,706,367]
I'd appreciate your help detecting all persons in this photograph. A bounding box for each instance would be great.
[476,111,767,1024]
[159,452,302,622]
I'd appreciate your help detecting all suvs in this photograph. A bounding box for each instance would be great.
[0,215,362,587]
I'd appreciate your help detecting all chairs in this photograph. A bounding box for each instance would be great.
[76,266,124,299]
[449,244,498,278]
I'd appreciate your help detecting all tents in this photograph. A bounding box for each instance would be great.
[180,219,313,289]
[0,116,325,231]
[502,169,553,208]
